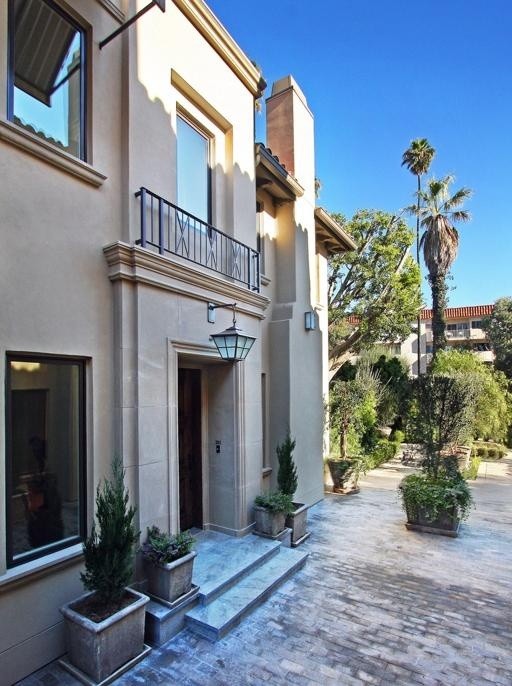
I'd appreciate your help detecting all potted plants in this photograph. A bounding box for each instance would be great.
[252,489,291,540]
[328,379,364,494]
[56,456,151,685]
[141,525,200,610]
[276,434,310,547]
[398,376,474,538]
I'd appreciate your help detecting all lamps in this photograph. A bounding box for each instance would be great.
[208,302,258,367]
[304,311,315,331]
